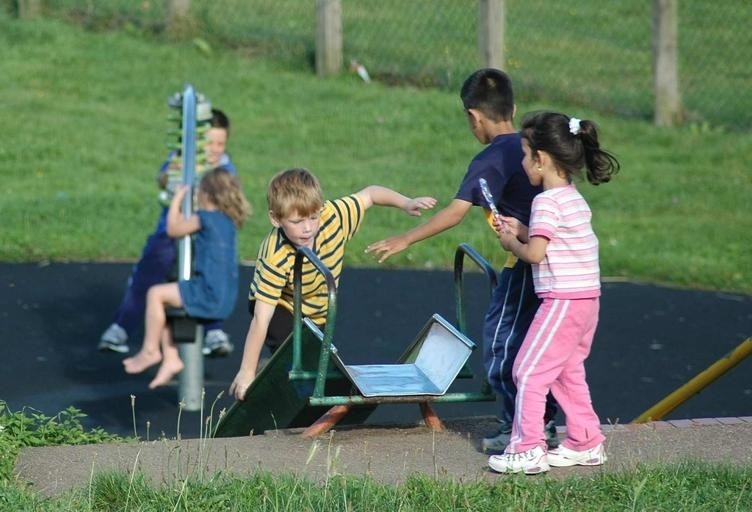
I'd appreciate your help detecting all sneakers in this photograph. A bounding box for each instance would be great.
[488,446,549,475]
[497,420,512,433]
[203,329,233,358]
[545,421,606,466]
[99,323,131,354]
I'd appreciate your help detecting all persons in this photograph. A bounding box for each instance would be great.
[97,107,233,358]
[485,110,621,472]
[121,167,254,389]
[226,166,438,402]
[364,68,563,454]
